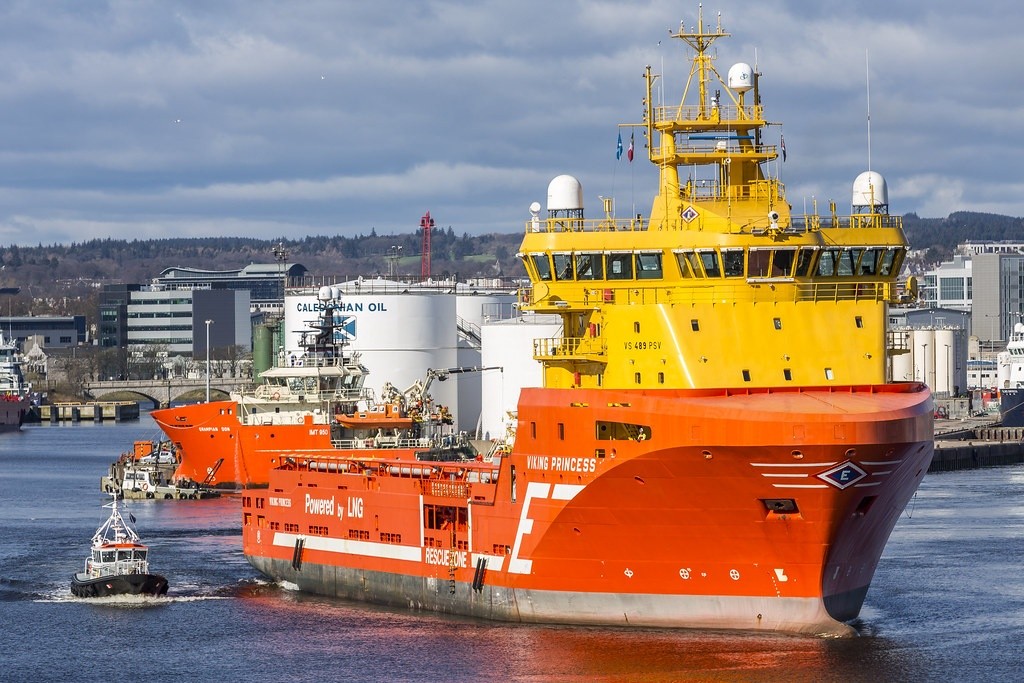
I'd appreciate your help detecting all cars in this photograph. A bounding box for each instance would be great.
[138,451,177,464]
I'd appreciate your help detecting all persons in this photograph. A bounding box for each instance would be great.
[563,262,573,280]
[637,427,647,441]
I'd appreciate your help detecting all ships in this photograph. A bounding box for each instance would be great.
[243,4,934,640]
[0,336,23,430]
[148,285,486,491]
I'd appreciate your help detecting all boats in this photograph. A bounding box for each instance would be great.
[71,467,170,600]
[89,457,226,500]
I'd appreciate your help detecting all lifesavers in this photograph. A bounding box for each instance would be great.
[297,416,304,423]
[274,392,280,398]
[143,483,149,490]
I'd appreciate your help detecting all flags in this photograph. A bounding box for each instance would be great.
[616,128,623,162]
[781,134,787,162]
[627,132,634,163]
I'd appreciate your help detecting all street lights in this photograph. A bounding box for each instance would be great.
[203,317,216,403]
